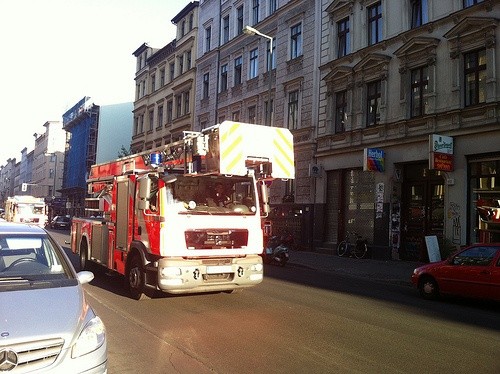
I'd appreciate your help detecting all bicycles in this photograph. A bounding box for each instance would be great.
[337,230,368,260]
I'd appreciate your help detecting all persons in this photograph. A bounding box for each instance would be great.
[214,182,230,206]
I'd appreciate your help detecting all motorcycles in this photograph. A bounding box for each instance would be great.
[262,235,290,267]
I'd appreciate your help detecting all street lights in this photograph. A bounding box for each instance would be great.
[242,25,272,127]
[42,151,57,198]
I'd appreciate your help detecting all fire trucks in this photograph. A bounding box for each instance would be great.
[4,195,49,228]
[69,119,296,300]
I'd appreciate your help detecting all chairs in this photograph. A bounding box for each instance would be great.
[0,248,38,273]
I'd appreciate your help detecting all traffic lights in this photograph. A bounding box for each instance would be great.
[21,183,27,191]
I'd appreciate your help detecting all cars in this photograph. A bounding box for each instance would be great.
[0,208,8,223]
[411,244,500,306]
[0,222,109,374]
[50,216,71,231]
